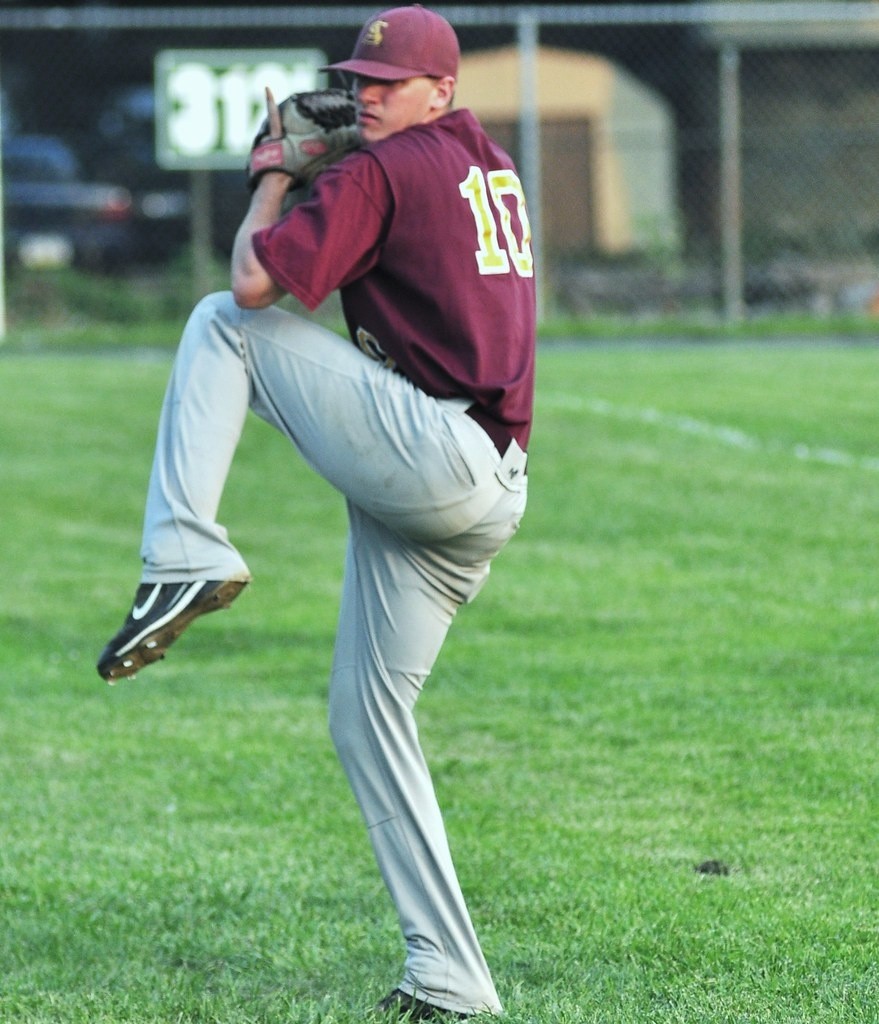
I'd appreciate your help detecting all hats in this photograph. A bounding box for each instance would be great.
[318,4,459,79]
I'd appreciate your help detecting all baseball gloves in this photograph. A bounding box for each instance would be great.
[246,86,356,192]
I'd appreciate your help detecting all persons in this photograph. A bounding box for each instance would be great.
[97,4,536,1024]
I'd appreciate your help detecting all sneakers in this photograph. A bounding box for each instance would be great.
[364,988,477,1024]
[97,582,246,682]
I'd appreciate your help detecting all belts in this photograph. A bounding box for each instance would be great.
[466,401,530,480]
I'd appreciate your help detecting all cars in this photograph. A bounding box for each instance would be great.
[0,127,193,269]
